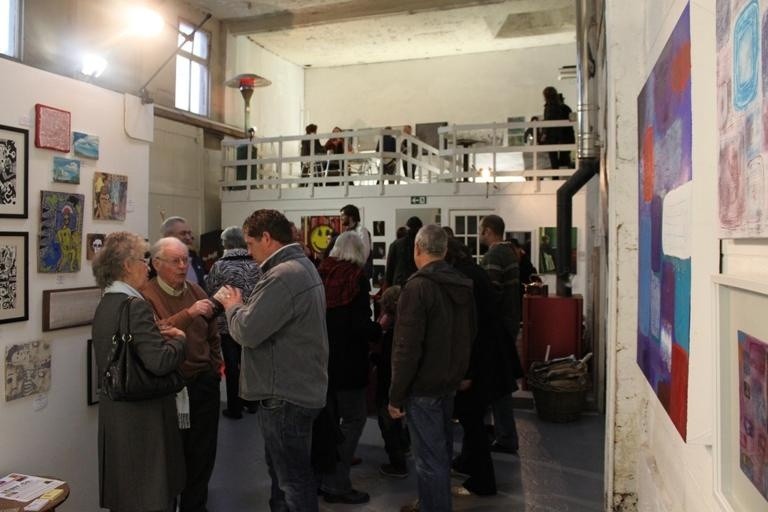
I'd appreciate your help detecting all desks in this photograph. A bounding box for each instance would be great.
[0,470,73,510]
[448,136,484,183]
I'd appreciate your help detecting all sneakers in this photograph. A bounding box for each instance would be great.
[451,485,473,496]
[380,463,408,479]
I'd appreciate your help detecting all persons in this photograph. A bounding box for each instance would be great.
[93,204,541,512]
[237,129,258,190]
[95,174,113,219]
[400,126,418,180]
[540,87,576,180]
[523,116,542,145]
[4,347,26,401]
[323,128,352,185]
[298,124,326,186]
[375,127,405,184]
[91,235,105,254]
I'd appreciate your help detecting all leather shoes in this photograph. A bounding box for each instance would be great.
[326,487,370,504]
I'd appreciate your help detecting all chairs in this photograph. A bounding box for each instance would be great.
[302,152,372,190]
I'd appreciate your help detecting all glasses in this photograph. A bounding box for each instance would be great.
[158,256,189,265]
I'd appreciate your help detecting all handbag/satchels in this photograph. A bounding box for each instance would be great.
[104,333,186,401]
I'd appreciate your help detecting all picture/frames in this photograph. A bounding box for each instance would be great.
[0,230,30,325]
[40,285,101,332]
[0,123,31,221]
[35,104,71,154]
[86,339,102,408]
[712,280,767,512]
